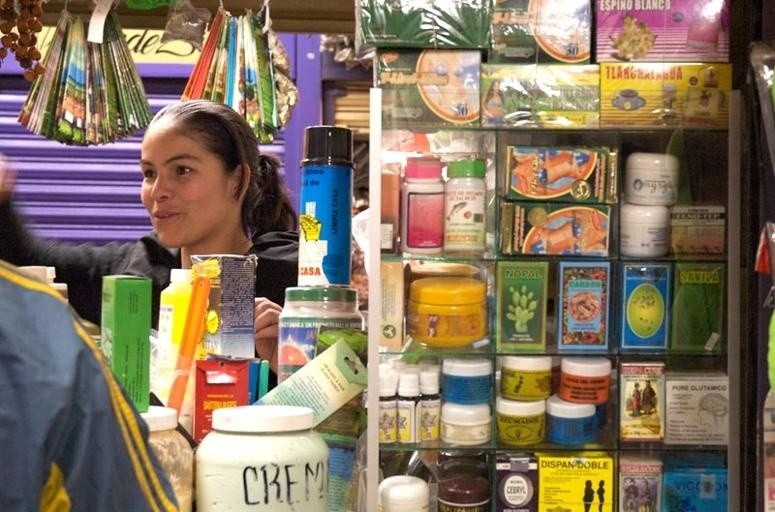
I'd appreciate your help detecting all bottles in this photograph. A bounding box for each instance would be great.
[401,157,490,261]
[195,404,332,511]
[380,358,441,445]
[138,404,194,511]
[296,127,356,287]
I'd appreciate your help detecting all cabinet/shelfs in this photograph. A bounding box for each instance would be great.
[363,80,759,508]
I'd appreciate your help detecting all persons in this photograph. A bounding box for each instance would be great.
[0,260,180,512]
[0,100,302,403]
